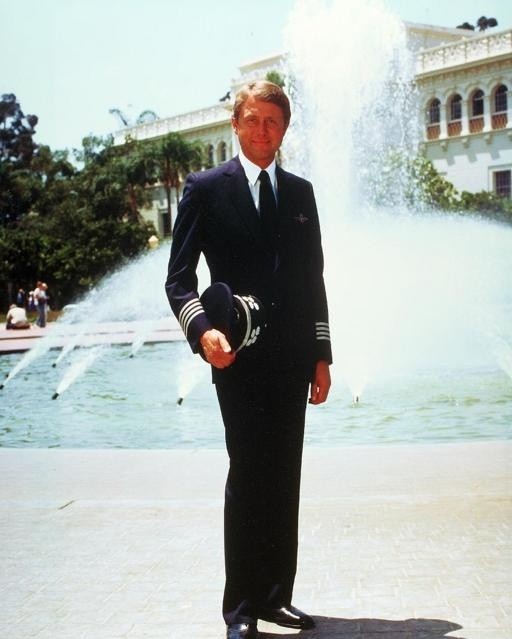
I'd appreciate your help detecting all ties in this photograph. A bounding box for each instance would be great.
[258,170,277,223]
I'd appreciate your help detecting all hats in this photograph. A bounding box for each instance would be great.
[198,283,266,363]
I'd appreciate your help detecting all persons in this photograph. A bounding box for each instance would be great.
[5,281,50,329]
[164,80,336,638]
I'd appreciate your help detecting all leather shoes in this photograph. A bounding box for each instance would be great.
[263,605,316,630]
[227,622,258,639]
